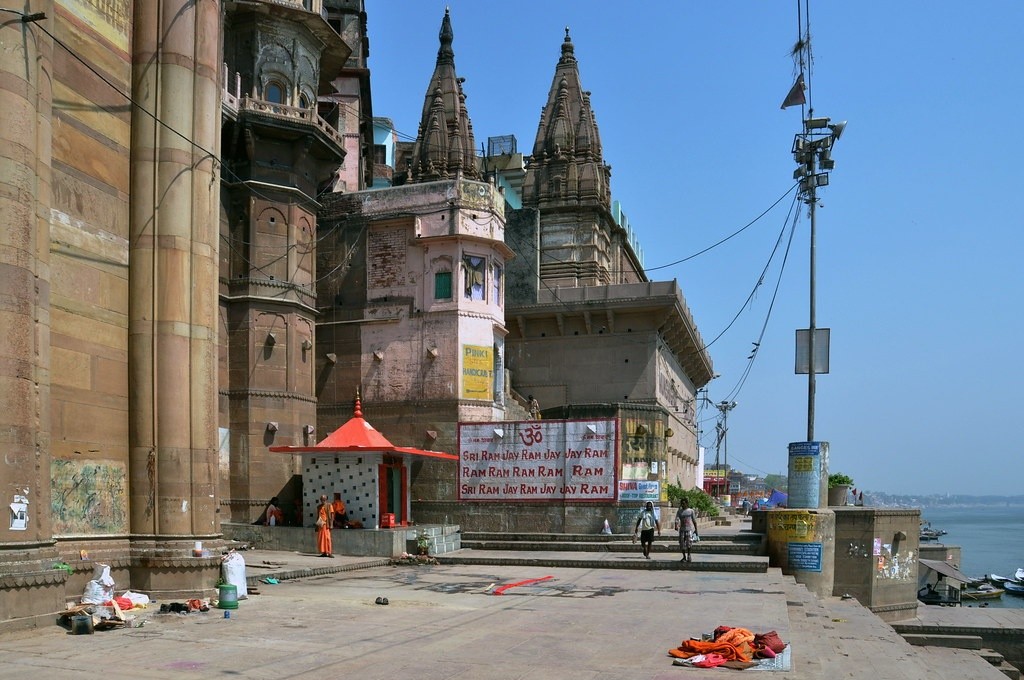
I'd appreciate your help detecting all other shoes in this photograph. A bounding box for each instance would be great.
[646,556,651,559]
[681,556,686,561]
[687,555,691,562]
[321,554,327,556]
[644,550,646,556]
[328,554,334,558]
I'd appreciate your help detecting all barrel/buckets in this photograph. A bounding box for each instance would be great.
[218,584,238,609]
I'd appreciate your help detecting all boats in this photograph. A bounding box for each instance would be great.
[962,567,1024,599]
[920,525,948,539]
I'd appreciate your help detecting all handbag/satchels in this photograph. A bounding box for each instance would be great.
[326,519,334,529]
[754,631,786,656]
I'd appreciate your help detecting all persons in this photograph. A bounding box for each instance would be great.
[674,498,698,563]
[266,498,283,526]
[316,495,335,558]
[294,497,303,525]
[635,501,661,559]
[743,498,751,518]
[753,500,759,510]
[332,494,350,529]
[527,394,541,419]
